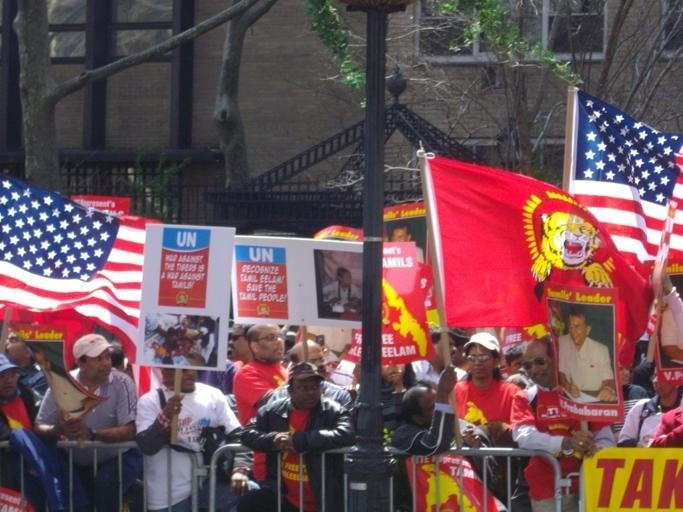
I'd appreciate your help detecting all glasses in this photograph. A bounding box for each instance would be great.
[466,355,489,362]
[523,357,545,369]
[256,334,283,341]
[229,333,246,341]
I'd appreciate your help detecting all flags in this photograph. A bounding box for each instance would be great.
[414,147,654,370]
[560,83,683,264]
[0,177,158,363]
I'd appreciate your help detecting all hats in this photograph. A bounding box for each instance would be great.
[462,332,501,353]
[289,361,324,381]
[0,353,19,372]
[72,334,114,361]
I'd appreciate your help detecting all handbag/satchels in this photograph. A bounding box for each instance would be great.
[198,424,234,476]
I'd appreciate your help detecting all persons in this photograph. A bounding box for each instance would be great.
[389,218,424,265]
[0,268,683,511]
